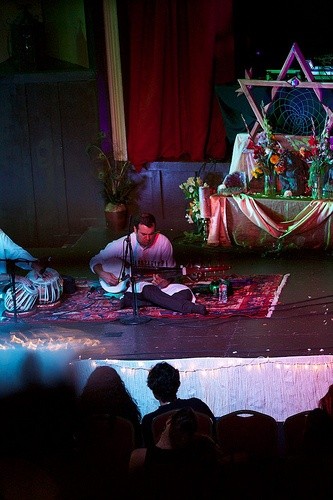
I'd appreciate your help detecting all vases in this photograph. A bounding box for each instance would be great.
[264,170,276,198]
[311,168,324,199]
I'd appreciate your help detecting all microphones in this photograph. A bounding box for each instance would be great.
[38,255,53,277]
[88,282,99,294]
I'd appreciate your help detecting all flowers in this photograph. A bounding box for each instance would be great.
[179,100,333,246]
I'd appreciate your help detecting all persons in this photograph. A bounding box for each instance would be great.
[0,360,333,500]
[0,227,55,322]
[88,213,208,317]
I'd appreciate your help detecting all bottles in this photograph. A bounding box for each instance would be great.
[219,279,227,302]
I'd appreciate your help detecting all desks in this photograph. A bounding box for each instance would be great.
[206,193,333,250]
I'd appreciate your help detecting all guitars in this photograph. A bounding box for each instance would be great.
[100,257,198,293]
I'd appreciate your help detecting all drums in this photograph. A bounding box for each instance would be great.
[25,267,63,304]
[3,280,38,311]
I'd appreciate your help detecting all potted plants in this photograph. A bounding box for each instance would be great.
[86,132,143,232]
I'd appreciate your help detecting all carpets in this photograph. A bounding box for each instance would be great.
[0,273,291,324]
